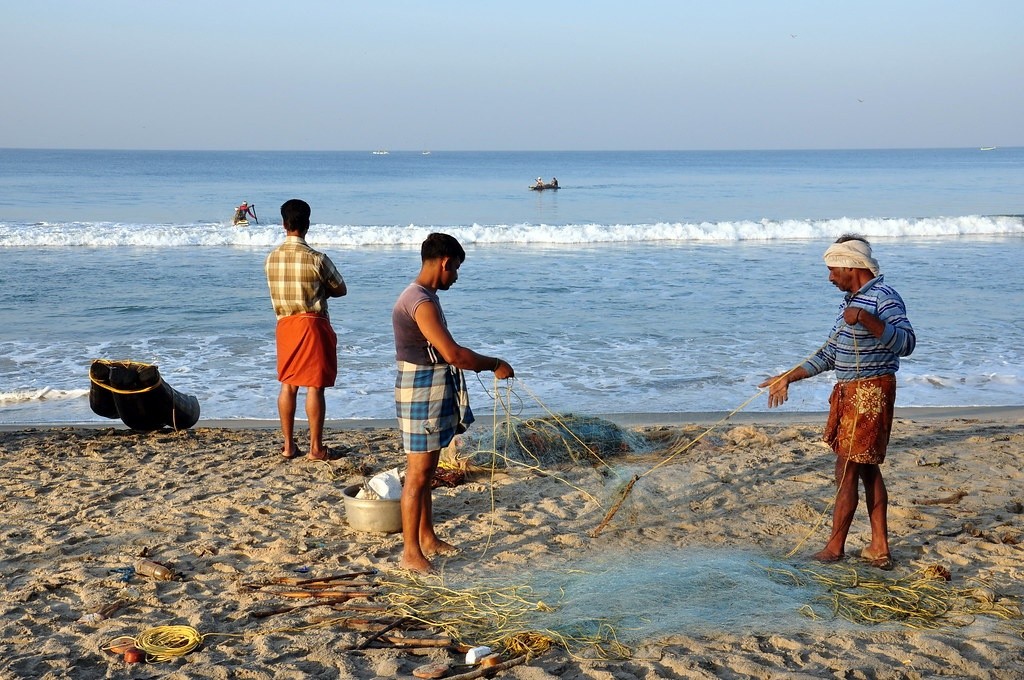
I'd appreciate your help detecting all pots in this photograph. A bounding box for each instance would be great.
[342,482,404,532]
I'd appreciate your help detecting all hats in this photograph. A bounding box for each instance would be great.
[242,200,248,205]
[537,176,541,180]
[235,207,239,211]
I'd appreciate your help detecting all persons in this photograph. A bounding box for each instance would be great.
[550,177,558,187]
[757,233,917,565]
[263,198,348,462]
[392,231,515,576]
[535,177,544,187]
[233,200,256,226]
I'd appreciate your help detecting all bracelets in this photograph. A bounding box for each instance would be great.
[855,307,863,322]
[492,356,498,372]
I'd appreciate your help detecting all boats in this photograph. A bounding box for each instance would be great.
[374,152,390,155]
[86,361,200,434]
[422,152,431,155]
[981,147,996,151]
[230,219,250,228]
[528,184,562,190]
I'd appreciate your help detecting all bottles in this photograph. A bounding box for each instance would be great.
[135,559,174,581]
